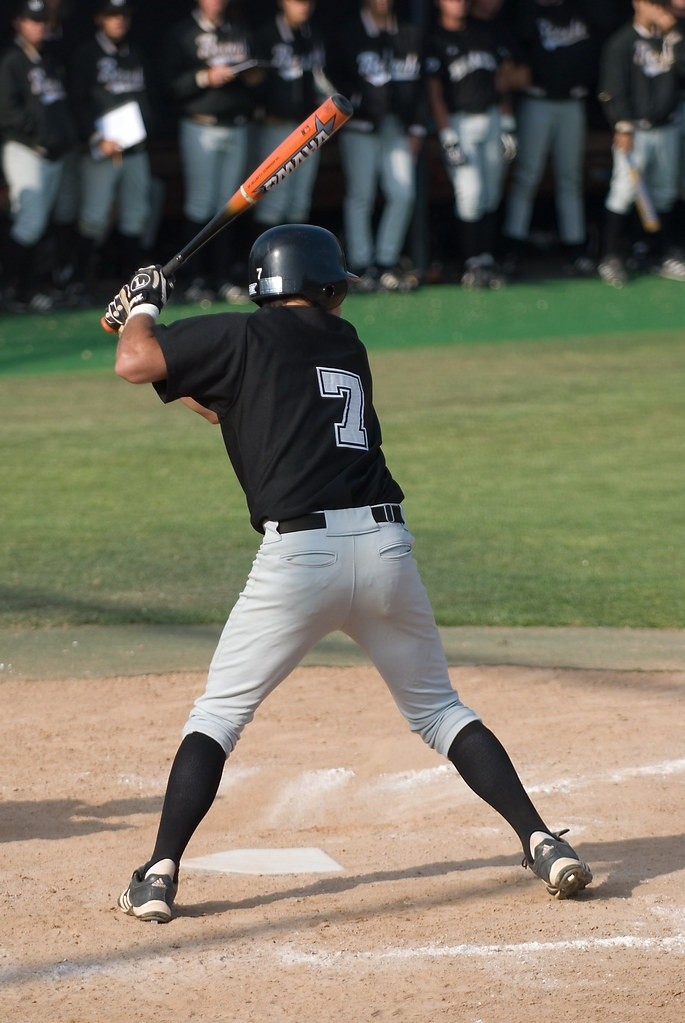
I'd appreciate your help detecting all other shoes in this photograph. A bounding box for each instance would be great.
[1,255,683,314]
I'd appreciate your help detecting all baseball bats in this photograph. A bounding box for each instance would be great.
[98,92,353,335]
[621,147,660,234]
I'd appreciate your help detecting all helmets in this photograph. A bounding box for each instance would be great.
[247,223,360,309]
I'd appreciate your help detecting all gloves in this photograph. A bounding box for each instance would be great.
[444,141,464,165]
[502,130,520,159]
[128,265,176,316]
[105,284,131,335]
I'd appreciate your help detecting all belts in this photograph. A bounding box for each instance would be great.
[276,506,404,534]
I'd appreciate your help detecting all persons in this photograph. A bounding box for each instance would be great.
[0,0,685,315]
[114,223,593,921]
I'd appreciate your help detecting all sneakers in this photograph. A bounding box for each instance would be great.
[118,865,180,922]
[521,829,593,900]
